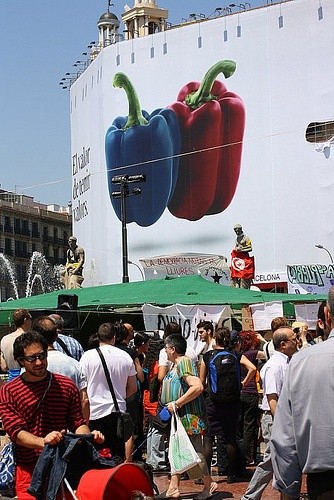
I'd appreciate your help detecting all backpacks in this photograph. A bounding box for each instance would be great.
[208,350,241,395]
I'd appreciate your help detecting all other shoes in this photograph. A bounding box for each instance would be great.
[153,466,169,474]
[227,469,253,483]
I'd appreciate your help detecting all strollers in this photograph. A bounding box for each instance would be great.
[50,434,155,500]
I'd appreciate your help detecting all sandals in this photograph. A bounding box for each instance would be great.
[194,483,219,500]
[155,489,181,500]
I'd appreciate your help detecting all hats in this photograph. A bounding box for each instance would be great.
[292,322,306,331]
[229,330,242,344]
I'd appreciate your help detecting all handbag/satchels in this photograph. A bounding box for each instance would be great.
[152,407,172,433]
[117,413,135,440]
[0,442,17,498]
[180,413,206,436]
[168,403,201,475]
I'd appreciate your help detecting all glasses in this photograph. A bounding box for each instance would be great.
[19,351,45,362]
[281,335,297,344]
[164,345,174,350]
[27,317,34,322]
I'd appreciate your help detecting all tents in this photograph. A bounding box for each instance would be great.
[0,273,328,329]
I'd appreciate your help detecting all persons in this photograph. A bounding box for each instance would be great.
[231,224,252,290]
[63,236,85,291]
[1,288,334,500]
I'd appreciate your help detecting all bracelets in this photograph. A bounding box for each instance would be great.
[175,403,181,408]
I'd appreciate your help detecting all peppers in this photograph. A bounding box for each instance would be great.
[104,72,180,227]
[168,59,246,220]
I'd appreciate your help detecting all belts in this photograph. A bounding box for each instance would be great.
[261,410,272,415]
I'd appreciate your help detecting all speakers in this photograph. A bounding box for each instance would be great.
[57,294,78,328]
